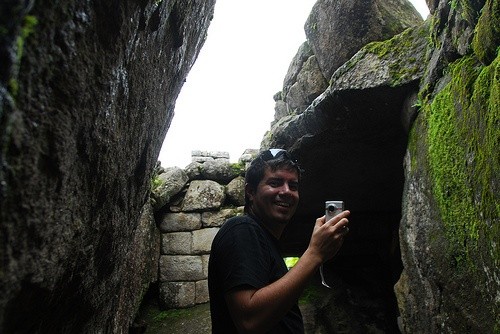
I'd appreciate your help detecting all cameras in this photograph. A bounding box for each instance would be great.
[323,199,344,227]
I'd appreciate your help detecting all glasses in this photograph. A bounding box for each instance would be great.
[256,148,298,166]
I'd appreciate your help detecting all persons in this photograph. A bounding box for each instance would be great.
[208,149,351,334]
